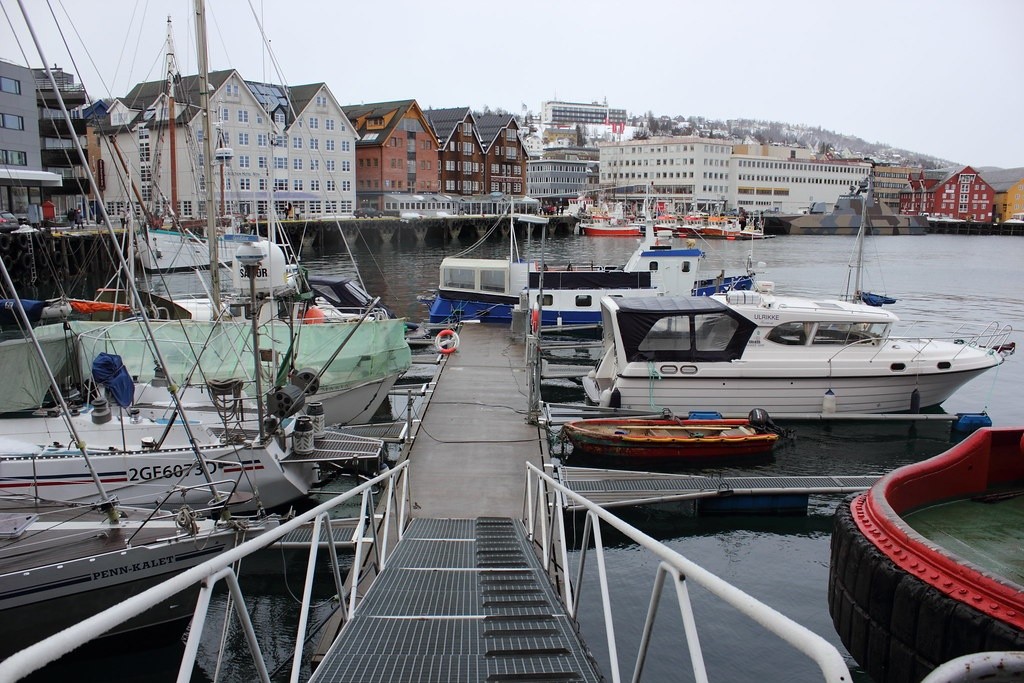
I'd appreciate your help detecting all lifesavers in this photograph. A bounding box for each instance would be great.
[435,342,459,352]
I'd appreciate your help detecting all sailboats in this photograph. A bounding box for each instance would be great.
[0,0,411,609]
[135,16,268,273]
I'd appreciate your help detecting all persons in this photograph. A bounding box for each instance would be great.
[68,208,84,229]
[120,209,130,228]
[460,207,535,214]
[583,202,587,210]
[535,205,564,216]
[283,201,292,219]
[258,208,265,218]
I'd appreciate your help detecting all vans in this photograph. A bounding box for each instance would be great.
[923,213,930,218]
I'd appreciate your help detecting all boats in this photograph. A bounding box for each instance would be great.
[790,171,930,236]
[583,196,1016,408]
[418,186,761,324]
[564,416,780,460]
[579,175,764,239]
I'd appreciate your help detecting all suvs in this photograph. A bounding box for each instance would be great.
[0,211,21,233]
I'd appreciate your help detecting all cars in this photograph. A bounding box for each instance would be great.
[353,207,385,219]
